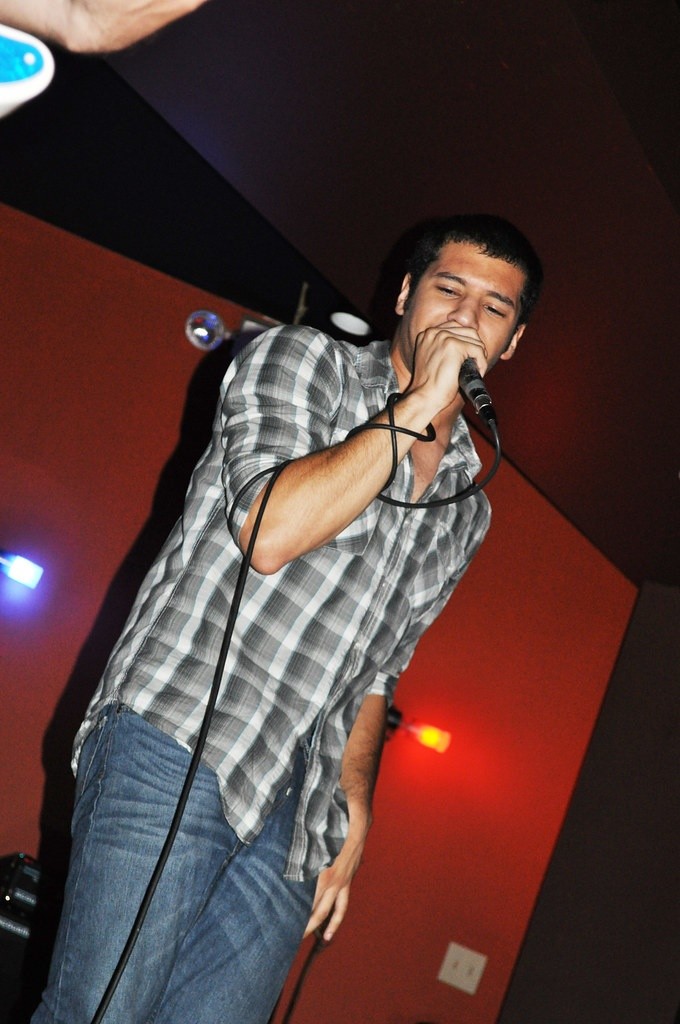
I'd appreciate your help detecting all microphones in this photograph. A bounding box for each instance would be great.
[459,359,497,428]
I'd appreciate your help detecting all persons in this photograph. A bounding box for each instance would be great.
[31,212,545,1024]
